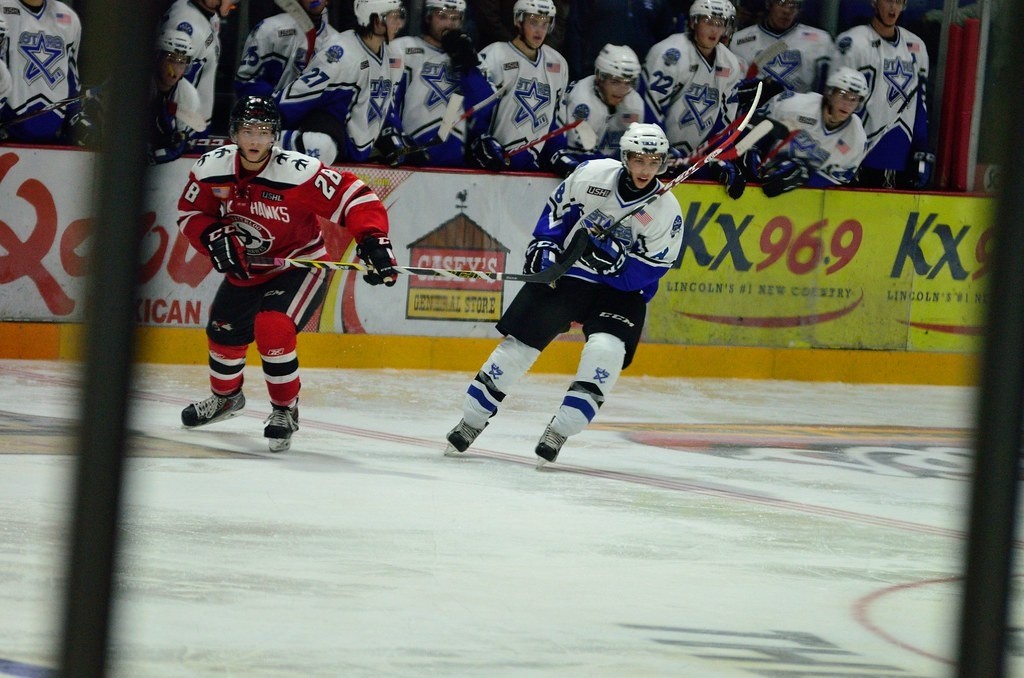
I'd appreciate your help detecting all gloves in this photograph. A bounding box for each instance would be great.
[763,159,808,196]
[468,134,507,172]
[906,147,937,186]
[355,235,398,287]
[523,240,563,289]
[441,28,478,70]
[376,125,406,167]
[577,220,627,276]
[69,107,100,148]
[717,158,745,200]
[204,224,247,273]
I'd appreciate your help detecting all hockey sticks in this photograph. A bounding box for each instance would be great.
[597,79,804,242]
[505,112,587,158]
[375,59,525,159]
[239,227,592,286]
[1,71,116,127]
[164,102,207,133]
[274,0,319,68]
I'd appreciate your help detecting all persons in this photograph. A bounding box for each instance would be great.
[233,0,430,168]
[642,0,835,199]
[0,0,102,146]
[739,66,869,199]
[563,40,681,177]
[446,122,684,461]
[110,0,239,163]
[381,0,578,179]
[176,93,399,439]
[826,0,936,191]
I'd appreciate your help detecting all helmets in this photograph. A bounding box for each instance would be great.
[155,28,194,64]
[826,65,868,97]
[620,122,668,174]
[425,0,467,19]
[514,0,556,32]
[690,0,736,21]
[355,0,400,26]
[228,94,280,133]
[594,43,641,80]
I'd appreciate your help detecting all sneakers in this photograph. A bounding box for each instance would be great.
[535,415,567,470]
[180,373,246,429]
[263,395,299,452]
[443,418,488,455]
